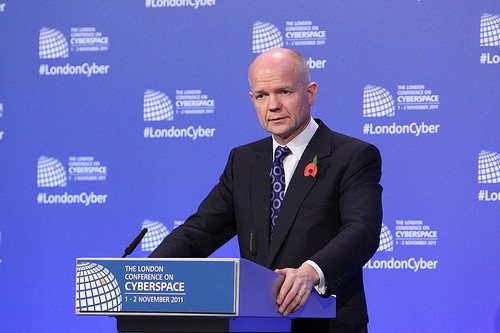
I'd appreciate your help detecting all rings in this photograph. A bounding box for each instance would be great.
[297,293,302,298]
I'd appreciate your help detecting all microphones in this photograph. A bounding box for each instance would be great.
[121,228,148,258]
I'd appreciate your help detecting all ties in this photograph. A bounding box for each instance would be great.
[270,146,292,239]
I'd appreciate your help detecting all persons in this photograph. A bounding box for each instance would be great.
[147,48,384,333]
